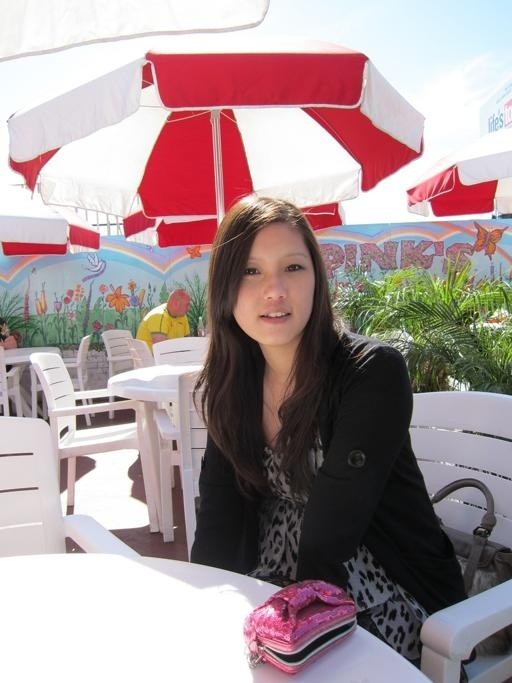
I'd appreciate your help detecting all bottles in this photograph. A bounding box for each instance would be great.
[197,316,205,337]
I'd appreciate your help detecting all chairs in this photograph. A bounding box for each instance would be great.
[27,332,97,428]
[27,350,161,535]
[0,345,24,418]
[0,412,140,555]
[399,387,511,683]
[125,336,155,370]
[152,368,232,555]
[100,329,144,419]
[151,336,212,363]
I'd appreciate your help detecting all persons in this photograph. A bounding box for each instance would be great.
[136,288,191,353]
[191,195,476,682]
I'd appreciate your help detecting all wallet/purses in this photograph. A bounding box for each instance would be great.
[244,578,358,675]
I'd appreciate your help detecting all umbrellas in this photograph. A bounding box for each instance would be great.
[407,126,512,217]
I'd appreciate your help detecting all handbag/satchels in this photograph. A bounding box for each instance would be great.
[437,520,512,658]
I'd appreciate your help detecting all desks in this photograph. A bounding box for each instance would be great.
[0,552,434,683]
[4,343,60,401]
[103,360,214,541]
